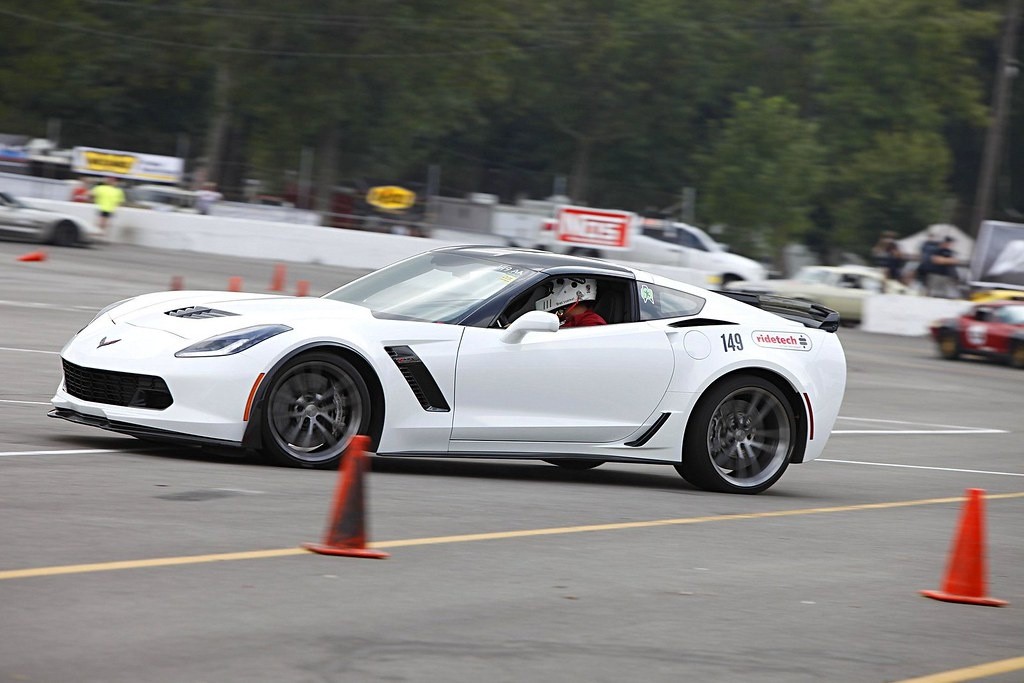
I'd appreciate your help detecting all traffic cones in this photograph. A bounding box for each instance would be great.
[303,434,387,558]
[917,487,1010,607]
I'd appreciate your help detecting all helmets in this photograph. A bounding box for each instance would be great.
[536,278,598,314]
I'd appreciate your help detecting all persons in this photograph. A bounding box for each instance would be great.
[534,276,608,331]
[68,172,126,238]
[865,225,974,303]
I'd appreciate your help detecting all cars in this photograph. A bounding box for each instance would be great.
[927,298,1023,366]
[43,239,848,494]
[0,133,1024,326]
